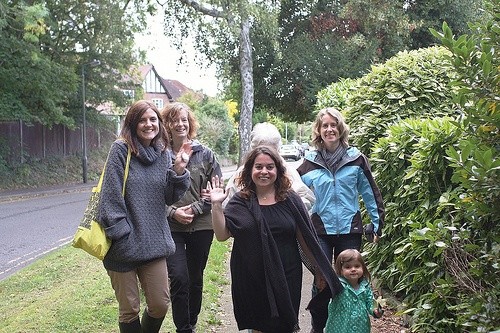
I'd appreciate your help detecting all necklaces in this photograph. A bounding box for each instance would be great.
[257,186,275,200]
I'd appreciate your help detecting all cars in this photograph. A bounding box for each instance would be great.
[280,140,309,161]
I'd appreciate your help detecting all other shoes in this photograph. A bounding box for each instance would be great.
[192,325,197,333]
[310,329,324,333]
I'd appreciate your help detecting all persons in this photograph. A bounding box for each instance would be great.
[95,99,193,333]
[315,248,388,333]
[160,102,226,333]
[293,107,385,333]
[207,121,326,333]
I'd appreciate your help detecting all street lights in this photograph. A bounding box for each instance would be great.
[81,61,101,183]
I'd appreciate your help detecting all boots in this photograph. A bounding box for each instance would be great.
[117,309,165,333]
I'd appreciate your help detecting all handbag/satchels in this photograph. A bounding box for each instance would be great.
[71,139,133,262]
